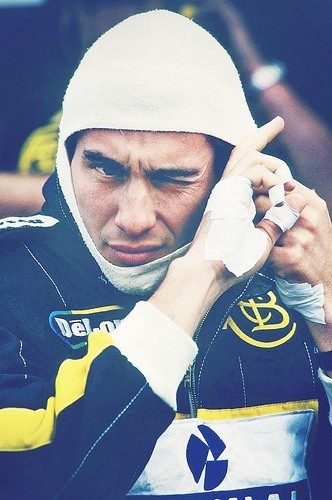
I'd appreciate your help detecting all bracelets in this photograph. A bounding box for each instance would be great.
[248,59,288,98]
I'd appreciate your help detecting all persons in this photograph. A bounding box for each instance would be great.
[0,9,332,499]
[0,1,332,224]
